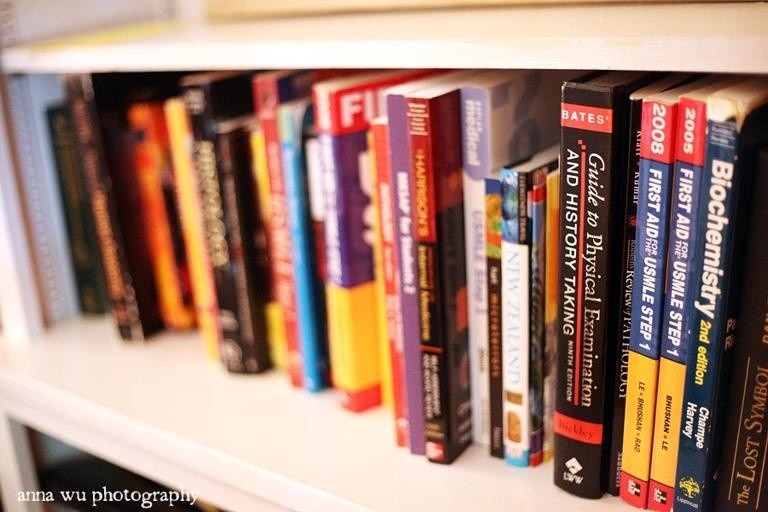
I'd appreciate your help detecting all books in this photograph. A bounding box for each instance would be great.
[0,71,768,512]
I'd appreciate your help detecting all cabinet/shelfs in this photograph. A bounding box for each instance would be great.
[2,3,768,511]
